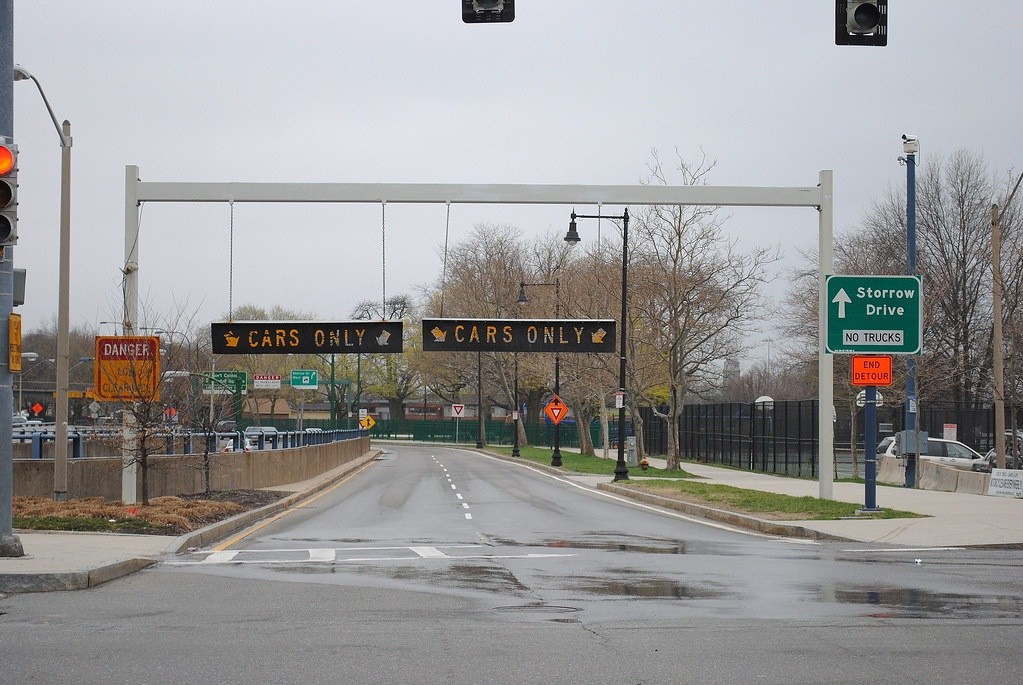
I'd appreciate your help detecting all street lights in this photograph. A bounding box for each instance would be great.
[161,369,243,449]
[155,331,194,431]
[762,338,774,395]
[141,327,175,429]
[564,207,630,483]
[19,358,55,415]
[515,278,564,466]
[14,64,72,501]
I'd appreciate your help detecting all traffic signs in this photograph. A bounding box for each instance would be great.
[289,370,319,390]
[824,275,923,355]
[201,370,248,395]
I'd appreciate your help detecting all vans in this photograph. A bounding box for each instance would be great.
[876,436,985,477]
[215,421,236,440]
[12,414,28,427]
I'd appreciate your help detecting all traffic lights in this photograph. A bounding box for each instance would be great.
[0,144,19,246]
[831,0,889,45]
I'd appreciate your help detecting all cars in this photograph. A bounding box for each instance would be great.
[222,438,258,453]
[242,426,281,446]
